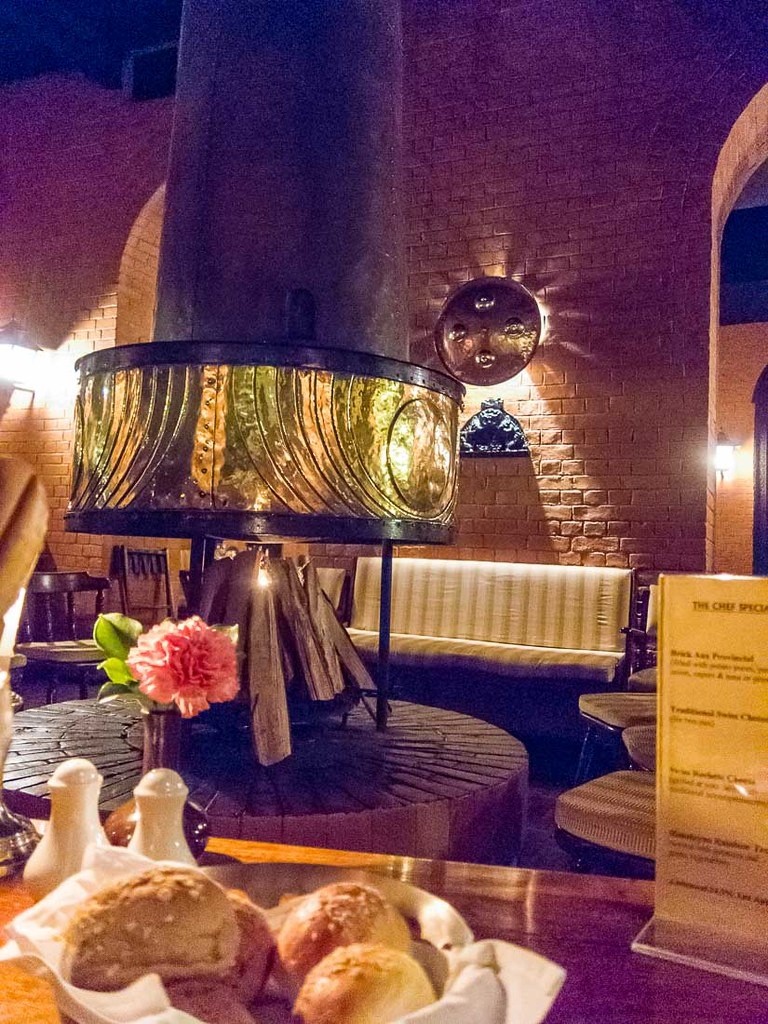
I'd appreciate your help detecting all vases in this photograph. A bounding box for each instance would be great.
[105,706,211,874]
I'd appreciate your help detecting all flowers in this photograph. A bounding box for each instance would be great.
[92,611,244,719]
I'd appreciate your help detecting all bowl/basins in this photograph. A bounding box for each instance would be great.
[194,862,476,970]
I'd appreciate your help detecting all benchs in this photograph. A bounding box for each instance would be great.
[315,567,352,624]
[344,556,651,738]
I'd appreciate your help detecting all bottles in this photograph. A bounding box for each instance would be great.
[23,758,103,903]
[127,769,201,871]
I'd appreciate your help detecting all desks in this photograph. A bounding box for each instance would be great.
[0,699,527,870]
[0,835,768,1024]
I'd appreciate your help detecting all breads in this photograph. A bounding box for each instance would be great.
[61,867,438,1024]
[0,457,51,638]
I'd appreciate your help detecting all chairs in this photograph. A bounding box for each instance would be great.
[555,627,655,881]
[19,571,113,700]
